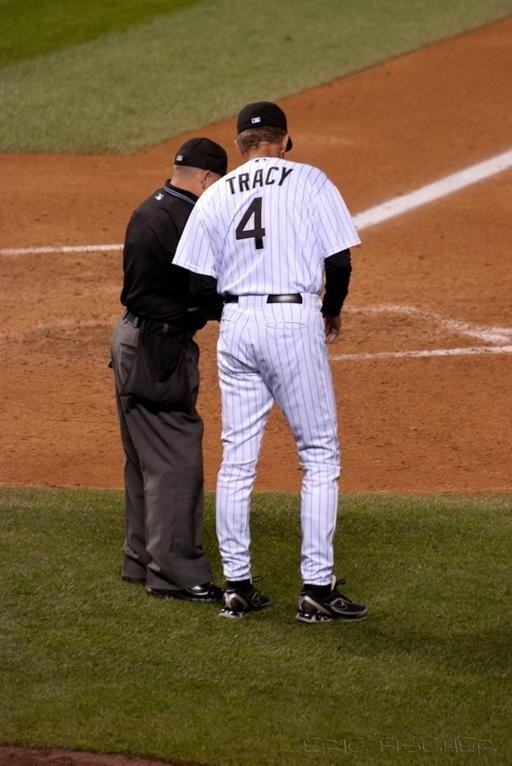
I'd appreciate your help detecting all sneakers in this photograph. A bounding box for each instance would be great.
[220,576,273,618]
[297,578,368,623]
[146,581,224,602]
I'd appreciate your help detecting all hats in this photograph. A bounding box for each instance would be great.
[174,138,227,176]
[236,101,292,152]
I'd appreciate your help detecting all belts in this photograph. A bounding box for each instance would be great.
[222,292,302,304]
[126,311,175,333]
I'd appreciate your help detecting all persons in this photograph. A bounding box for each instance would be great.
[171,101,369,624]
[108,136,228,602]
